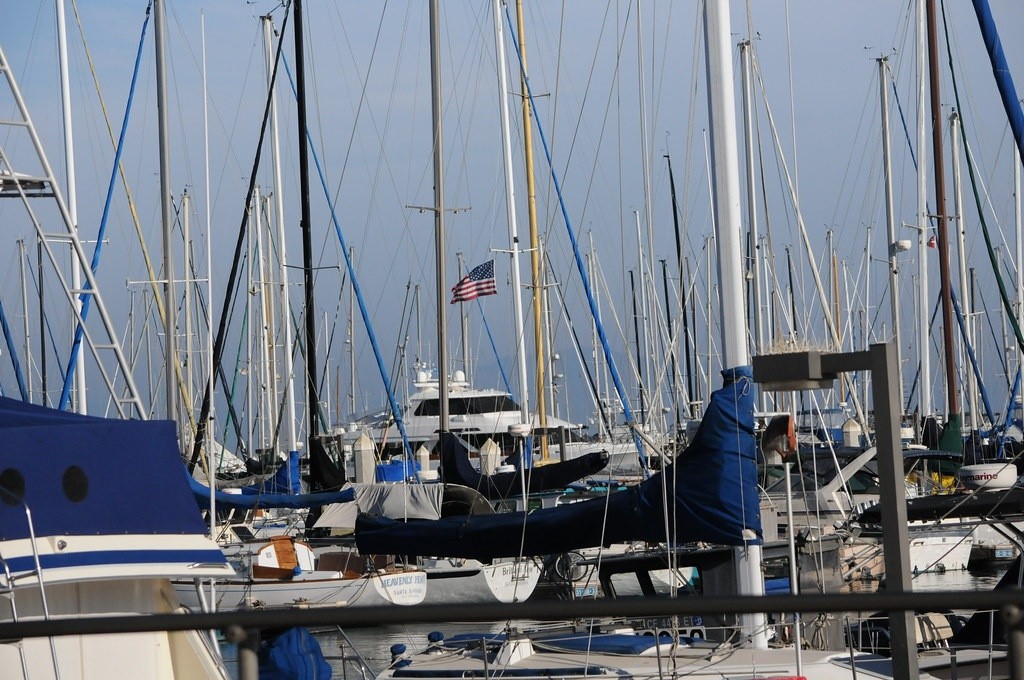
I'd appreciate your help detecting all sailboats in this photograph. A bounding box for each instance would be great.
[0,0,1024,680]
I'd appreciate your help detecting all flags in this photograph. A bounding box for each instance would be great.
[449,258,498,304]
